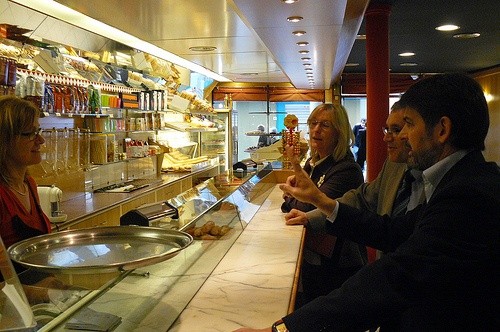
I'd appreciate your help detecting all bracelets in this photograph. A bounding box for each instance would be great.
[272,319,289,332]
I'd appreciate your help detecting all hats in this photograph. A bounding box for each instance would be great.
[270,127,277,133]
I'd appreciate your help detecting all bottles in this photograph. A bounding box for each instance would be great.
[38,127,90,175]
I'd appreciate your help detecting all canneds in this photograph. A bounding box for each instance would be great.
[136,91,161,112]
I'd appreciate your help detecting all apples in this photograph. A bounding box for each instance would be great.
[128,139,144,146]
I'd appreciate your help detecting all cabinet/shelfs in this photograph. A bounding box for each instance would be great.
[0,22,222,229]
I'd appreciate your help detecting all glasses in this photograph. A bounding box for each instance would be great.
[16,128,42,140]
[309,120,333,129]
[382,127,401,135]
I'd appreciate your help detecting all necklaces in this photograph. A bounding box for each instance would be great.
[2,175,27,196]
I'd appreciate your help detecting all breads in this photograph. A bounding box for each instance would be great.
[185,221,230,237]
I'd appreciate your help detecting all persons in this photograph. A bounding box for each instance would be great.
[234,70,500,332]
[0,95,55,284]
[285,100,427,263]
[280,103,369,303]
[257,125,277,147]
[353,119,367,172]
